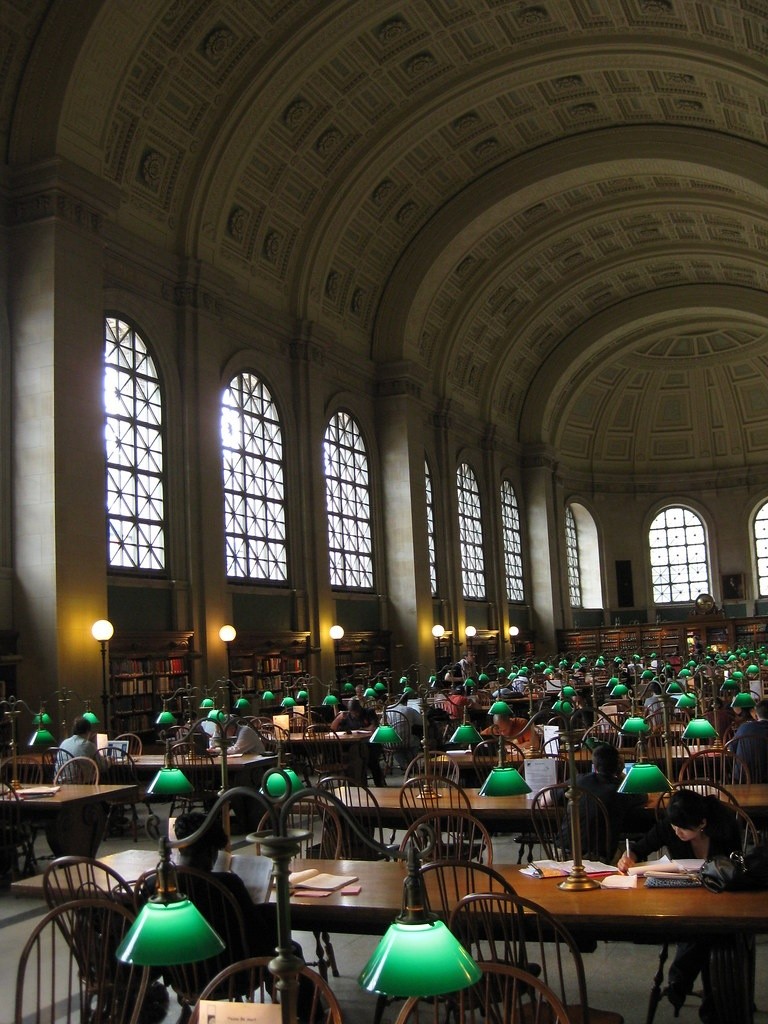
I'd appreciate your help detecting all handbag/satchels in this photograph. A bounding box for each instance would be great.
[699,844,768,894]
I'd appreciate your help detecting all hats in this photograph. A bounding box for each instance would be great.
[176,812,228,850]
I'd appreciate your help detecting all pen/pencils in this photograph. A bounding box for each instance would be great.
[625,838,629,858]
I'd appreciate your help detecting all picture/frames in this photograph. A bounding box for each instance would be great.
[719,571,749,602]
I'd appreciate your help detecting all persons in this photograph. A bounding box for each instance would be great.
[177,713,265,755]
[538,744,649,862]
[329,685,423,770]
[444,650,587,720]
[137,808,307,1002]
[480,691,594,749]
[644,669,710,725]
[691,636,703,657]
[607,662,633,689]
[53,718,114,785]
[702,698,768,784]
[616,788,742,1024]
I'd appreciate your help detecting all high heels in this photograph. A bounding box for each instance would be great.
[668,988,686,1017]
[698,1003,715,1024]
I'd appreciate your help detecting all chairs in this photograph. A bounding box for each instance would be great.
[0,690,768,1024]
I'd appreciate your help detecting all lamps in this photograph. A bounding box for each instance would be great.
[144,709,309,849]
[114,767,482,1024]
[396,644,768,690]
[465,625,477,651]
[31,684,102,741]
[198,676,253,737]
[280,674,341,738]
[509,626,519,665]
[92,620,115,741]
[219,624,236,713]
[156,684,226,761]
[432,625,445,671]
[261,671,309,712]
[330,625,344,699]
[0,695,59,793]
[478,697,674,892]
[343,666,387,691]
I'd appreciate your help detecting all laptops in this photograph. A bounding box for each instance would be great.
[471,734,511,756]
[546,680,562,690]
[590,760,637,776]
[106,740,130,762]
[669,693,684,714]
[310,705,334,724]
[404,699,422,714]
[182,732,218,758]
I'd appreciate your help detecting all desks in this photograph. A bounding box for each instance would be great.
[427,741,736,787]
[228,726,375,783]
[537,721,689,739]
[10,849,768,945]
[0,781,138,870]
[0,752,291,840]
[291,786,768,831]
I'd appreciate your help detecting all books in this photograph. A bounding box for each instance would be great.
[335,651,389,680]
[524,758,557,800]
[711,623,768,651]
[273,868,358,891]
[211,850,274,905]
[518,858,705,888]
[566,629,678,658]
[106,658,190,732]
[231,658,306,709]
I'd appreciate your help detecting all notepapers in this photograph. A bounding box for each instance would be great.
[340,885,362,894]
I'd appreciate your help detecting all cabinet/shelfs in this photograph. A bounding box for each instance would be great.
[334,629,390,699]
[228,628,311,716]
[434,630,454,672]
[554,614,768,660]
[109,630,194,746]
[466,629,499,673]
